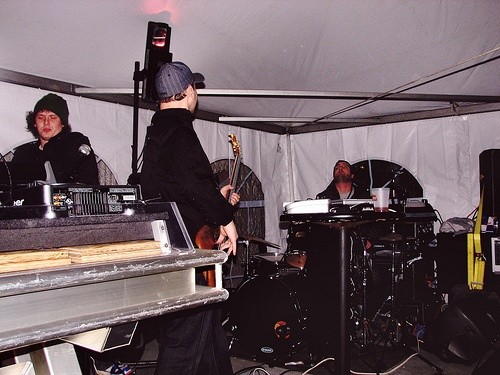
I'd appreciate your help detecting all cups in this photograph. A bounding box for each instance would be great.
[371,188,390,211]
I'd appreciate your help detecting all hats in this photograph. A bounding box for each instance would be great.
[34,93,68,125]
[155,62,205,97]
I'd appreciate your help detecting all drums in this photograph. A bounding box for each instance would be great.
[228,272,311,357]
[285,224,309,268]
[373,219,402,256]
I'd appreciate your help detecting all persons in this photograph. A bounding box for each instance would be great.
[317,160,371,249]
[10,93,99,187]
[141,62,240,375]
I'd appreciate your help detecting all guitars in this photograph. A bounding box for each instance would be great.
[195,132,244,287]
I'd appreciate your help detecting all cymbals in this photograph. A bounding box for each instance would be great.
[378,233,418,243]
[238,232,281,248]
